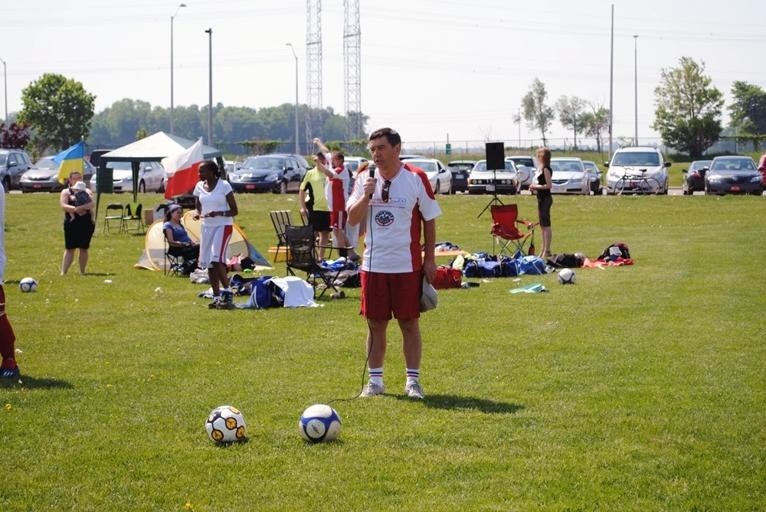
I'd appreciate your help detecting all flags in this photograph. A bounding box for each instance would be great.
[50,142,85,184]
[159,135,203,199]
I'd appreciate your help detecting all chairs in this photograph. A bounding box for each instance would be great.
[101,199,355,306]
[487,202,534,258]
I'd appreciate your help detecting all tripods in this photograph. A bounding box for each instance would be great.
[477,170,504,218]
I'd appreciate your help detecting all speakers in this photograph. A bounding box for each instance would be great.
[486,142,505,170]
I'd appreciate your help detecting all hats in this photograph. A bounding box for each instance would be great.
[71,181,86,191]
[419,275,439,314]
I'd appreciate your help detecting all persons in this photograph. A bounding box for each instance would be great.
[162,204,199,275]
[527,147,553,259]
[346,126,442,403]
[69,180,92,223]
[59,171,94,275]
[298,136,369,263]
[0,181,19,380]
[191,161,237,308]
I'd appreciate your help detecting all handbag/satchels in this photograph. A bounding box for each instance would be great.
[433,266,462,289]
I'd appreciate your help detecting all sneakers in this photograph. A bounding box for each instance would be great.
[359,381,385,398]
[208,295,233,311]
[0,365,21,383]
[405,382,425,400]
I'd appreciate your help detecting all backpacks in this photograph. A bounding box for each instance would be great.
[449,251,546,277]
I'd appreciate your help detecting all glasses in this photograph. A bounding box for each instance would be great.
[381,180,391,203]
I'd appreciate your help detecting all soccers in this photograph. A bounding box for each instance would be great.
[18,277,39,292]
[557,268,576,285]
[205,405,246,442]
[299,404,341,443]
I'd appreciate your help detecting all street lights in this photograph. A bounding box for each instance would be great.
[169,3,187,137]
[286,43,302,155]
[632,32,641,148]
[204,27,214,147]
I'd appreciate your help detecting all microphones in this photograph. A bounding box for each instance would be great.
[368,163,376,200]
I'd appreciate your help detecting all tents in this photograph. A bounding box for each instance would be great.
[131,208,271,271]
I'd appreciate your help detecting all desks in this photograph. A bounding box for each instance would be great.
[421,249,469,269]
